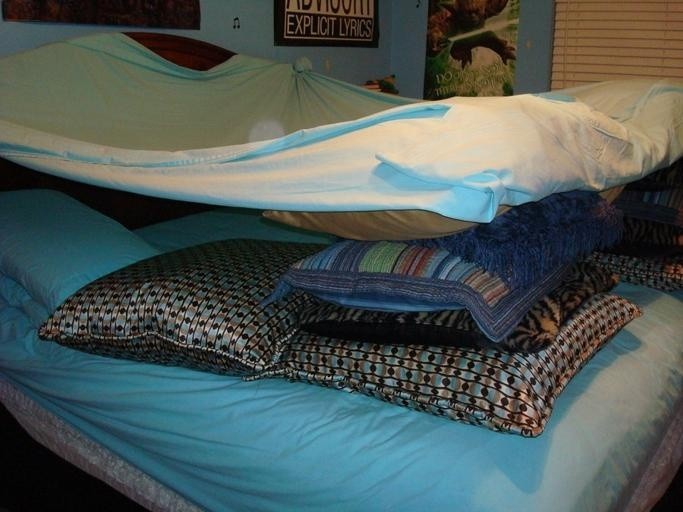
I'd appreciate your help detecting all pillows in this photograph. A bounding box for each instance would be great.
[609,203,683,250]
[300,264,621,352]
[583,250,683,291]
[260,239,568,345]
[39,239,325,377]
[242,292,641,437]
[263,206,511,243]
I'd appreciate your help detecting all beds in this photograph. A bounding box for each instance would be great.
[0,31,683,512]
